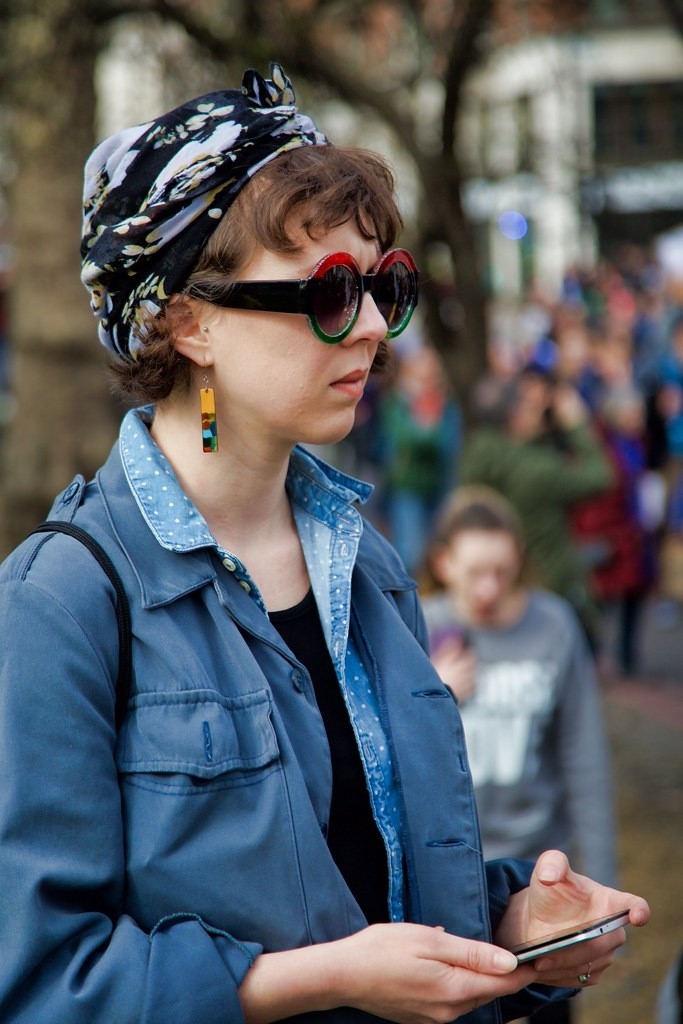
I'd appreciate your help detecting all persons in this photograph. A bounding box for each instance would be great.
[420,493,627,1024]
[1,62,650,1024]
[345,247,683,685]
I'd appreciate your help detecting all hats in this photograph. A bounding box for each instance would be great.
[80,60,335,365]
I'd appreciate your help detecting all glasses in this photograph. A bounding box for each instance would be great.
[182,248,422,344]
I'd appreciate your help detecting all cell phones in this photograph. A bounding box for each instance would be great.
[427,623,464,656]
[501,908,632,965]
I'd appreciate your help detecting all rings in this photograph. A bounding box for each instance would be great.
[578,962,591,983]
